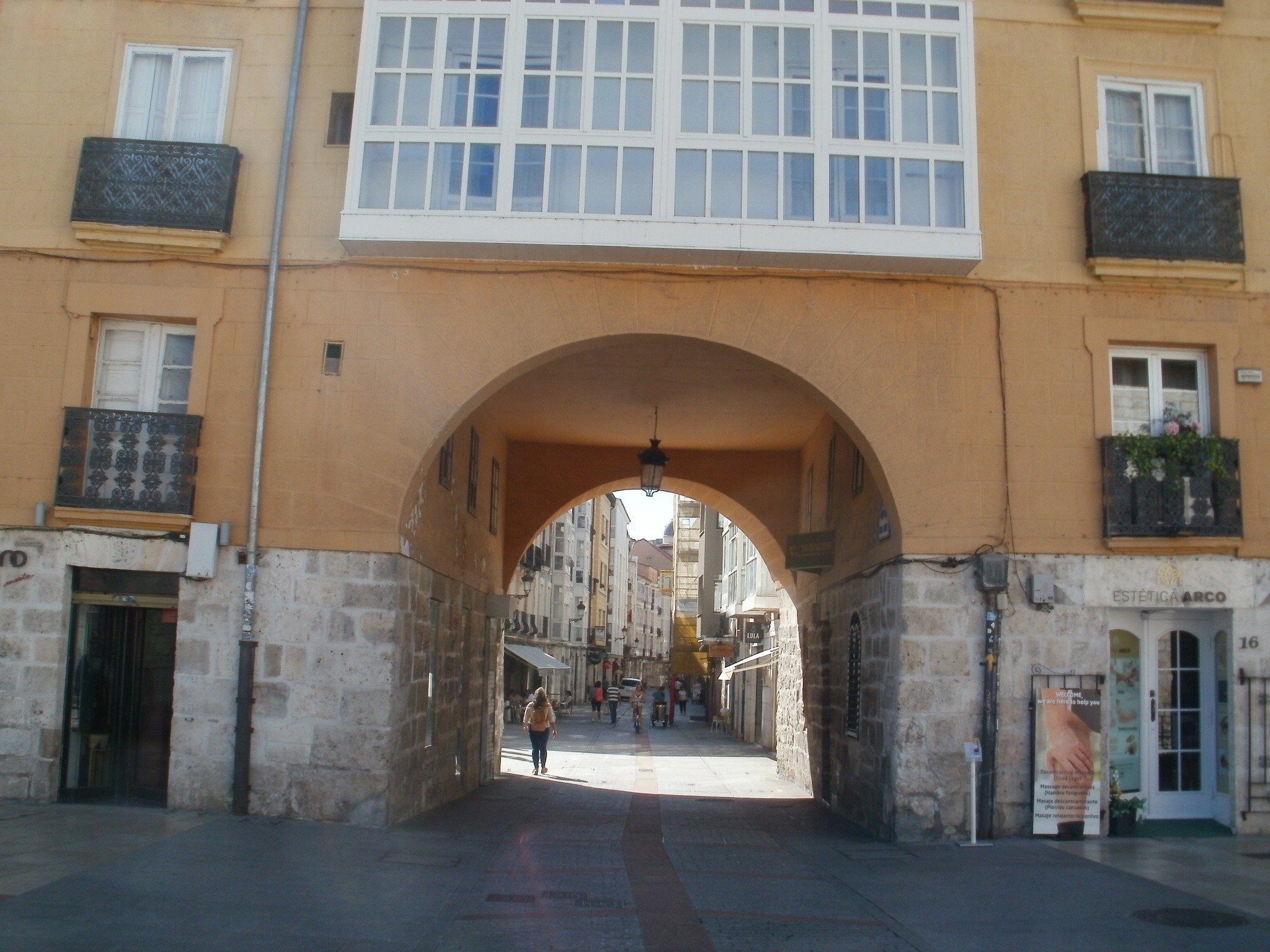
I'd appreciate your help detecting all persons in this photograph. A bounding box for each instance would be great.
[675,678,687,715]
[652,686,666,724]
[606,680,621,724]
[1041,686,1094,834]
[509,689,573,717]
[522,687,557,776]
[592,681,602,722]
[630,684,646,730]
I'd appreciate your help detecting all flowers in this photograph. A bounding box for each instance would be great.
[1113,421,1238,491]
[1110,770,1146,818]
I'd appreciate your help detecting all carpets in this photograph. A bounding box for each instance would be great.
[1136,819,1232,837]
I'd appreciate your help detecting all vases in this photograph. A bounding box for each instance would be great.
[1110,814,1136,838]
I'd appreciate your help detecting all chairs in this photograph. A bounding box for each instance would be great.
[511,699,572,723]
[711,708,730,733]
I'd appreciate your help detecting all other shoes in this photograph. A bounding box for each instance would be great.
[532,769,538,775]
[611,721,615,724]
[542,768,547,773]
[640,725,643,729]
[592,719,594,721]
[632,718,634,721]
[598,719,602,722]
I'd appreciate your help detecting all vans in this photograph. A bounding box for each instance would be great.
[619,677,642,700]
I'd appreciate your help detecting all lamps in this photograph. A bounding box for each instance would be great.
[662,651,669,658]
[219,520,231,545]
[507,570,533,600]
[569,601,586,623]
[35,502,45,527]
[615,626,628,642]
[631,638,639,648]
[638,407,669,497]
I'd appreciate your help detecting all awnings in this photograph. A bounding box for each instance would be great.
[504,644,571,678]
[718,646,779,681]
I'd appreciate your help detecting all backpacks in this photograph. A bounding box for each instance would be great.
[531,704,546,731]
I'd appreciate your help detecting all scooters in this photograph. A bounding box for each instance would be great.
[651,702,668,727]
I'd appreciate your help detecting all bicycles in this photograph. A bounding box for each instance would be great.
[632,701,643,733]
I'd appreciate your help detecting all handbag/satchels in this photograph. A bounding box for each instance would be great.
[604,704,609,715]
[590,688,596,700]
